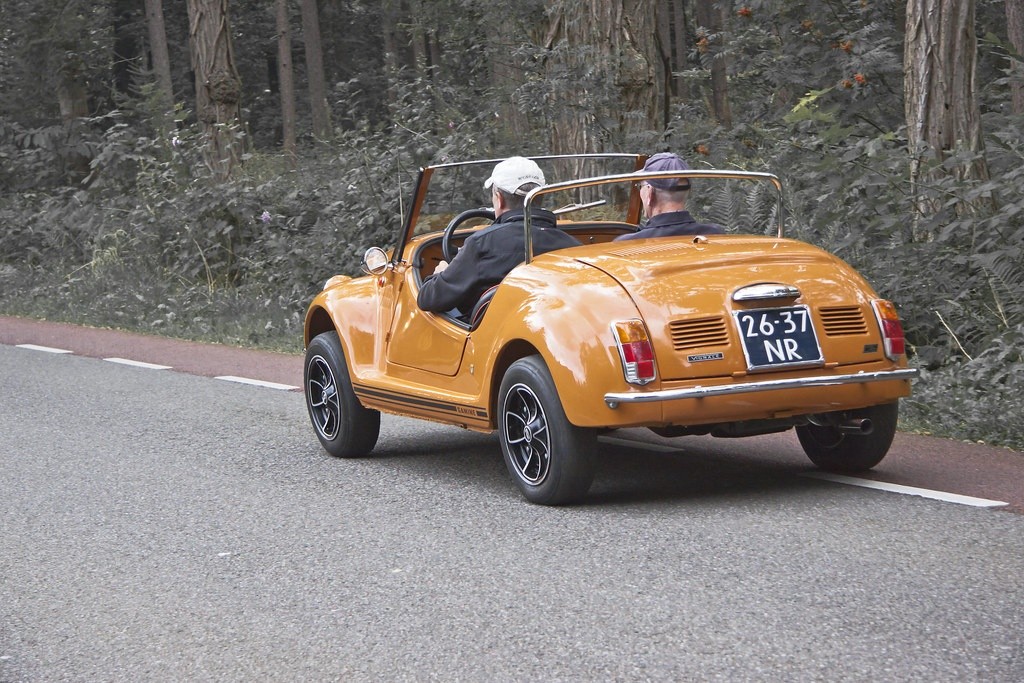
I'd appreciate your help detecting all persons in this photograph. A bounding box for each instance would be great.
[417,156,584,321]
[612,151,728,241]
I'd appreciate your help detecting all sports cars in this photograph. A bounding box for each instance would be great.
[302,152,923,507]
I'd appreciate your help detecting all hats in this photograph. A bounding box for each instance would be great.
[632,152,692,190]
[484,157,546,197]
